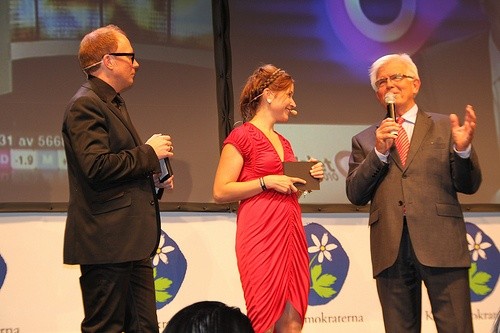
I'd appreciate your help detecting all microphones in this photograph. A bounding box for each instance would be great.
[385,92,398,136]
[290,110,297,116]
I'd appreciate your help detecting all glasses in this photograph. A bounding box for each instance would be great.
[375,73,414,88]
[101,53,135,63]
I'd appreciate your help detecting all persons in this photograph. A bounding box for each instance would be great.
[164,301,254,333]
[212,63,326,333]
[63,24,174,333]
[346,52,483,333]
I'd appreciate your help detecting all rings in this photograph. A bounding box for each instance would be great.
[170,146,172,152]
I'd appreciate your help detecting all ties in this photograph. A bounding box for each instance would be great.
[116,95,132,125]
[395,117,410,168]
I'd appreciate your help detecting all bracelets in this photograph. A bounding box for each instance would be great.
[260,177,267,191]
